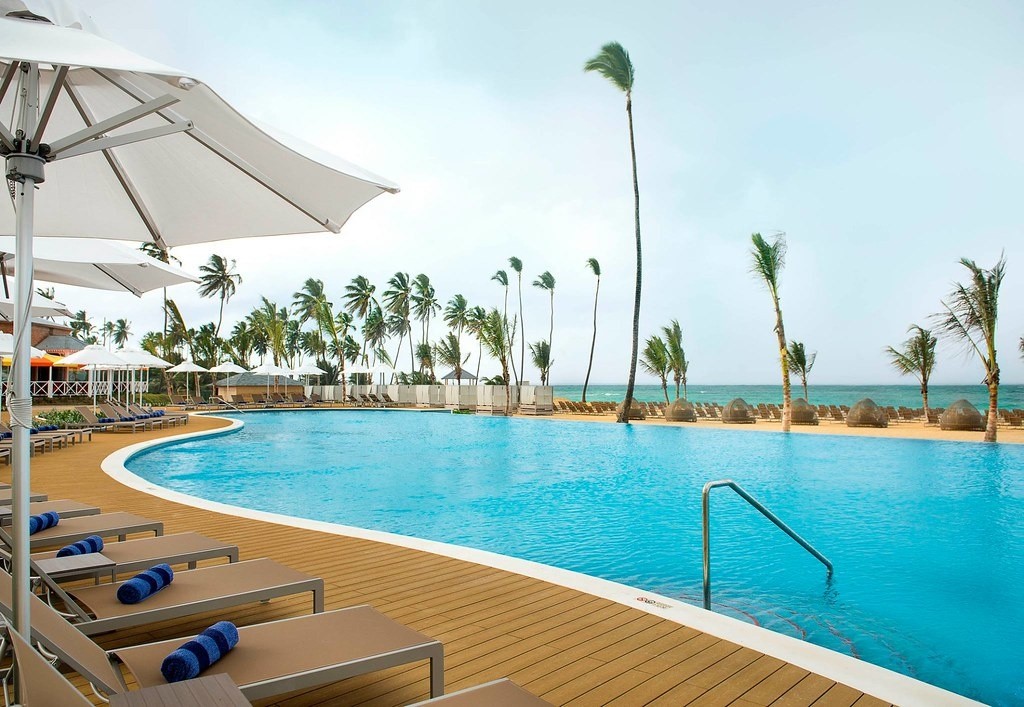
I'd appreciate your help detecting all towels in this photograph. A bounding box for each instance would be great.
[98,410,165,423]
[29,510,59,535]
[159,621,239,682]
[116,563,174,604]
[177,399,323,405]
[0,426,59,441]
[56,535,104,557]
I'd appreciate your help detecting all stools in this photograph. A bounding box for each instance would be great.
[110,672,254,707]
[0,507,13,515]
[33,551,116,589]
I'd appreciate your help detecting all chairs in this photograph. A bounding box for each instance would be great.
[75,402,190,433]
[346,392,414,410]
[166,390,339,412]
[0,480,101,527]
[0,527,325,647]
[0,533,238,594]
[0,612,558,707]
[0,564,445,707]
[0,424,91,466]
[0,512,164,557]
[550,400,1024,431]
